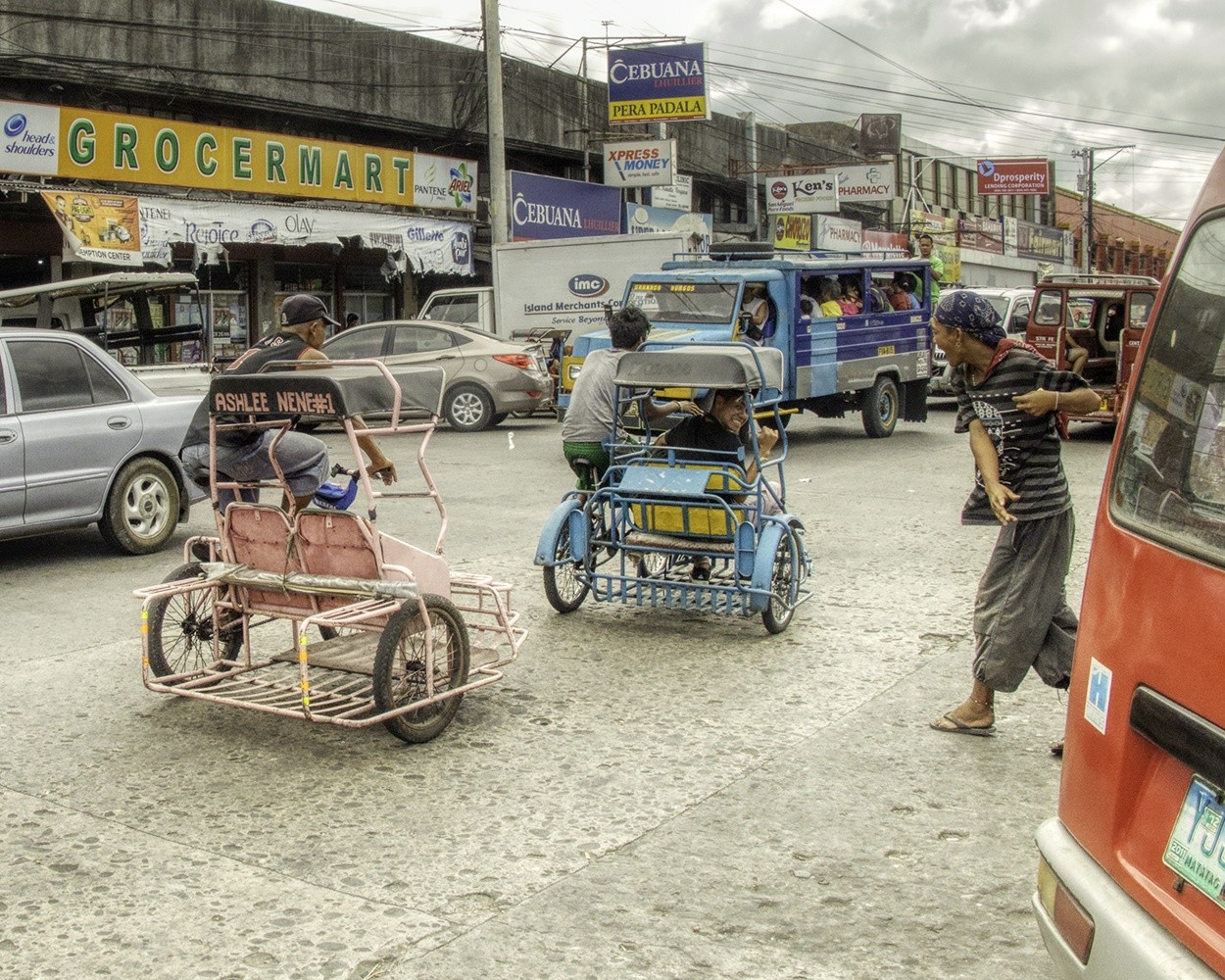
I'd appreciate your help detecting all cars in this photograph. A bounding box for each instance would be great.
[295,319,554,433]
[0,326,212,556]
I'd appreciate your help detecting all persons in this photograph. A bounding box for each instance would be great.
[179,293,396,561]
[928,291,1102,754]
[906,234,944,364]
[340,314,362,332]
[562,305,704,506]
[51,194,75,231]
[799,271,923,320]
[654,389,779,580]
[737,285,769,346]
[1040,303,1093,383]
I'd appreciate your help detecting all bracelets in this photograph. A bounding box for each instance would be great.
[967,695,998,714]
[672,400,682,414]
[1054,390,1062,413]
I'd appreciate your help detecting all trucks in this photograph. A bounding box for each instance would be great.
[414,229,710,358]
[557,243,935,438]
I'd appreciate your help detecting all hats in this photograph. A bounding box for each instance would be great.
[279,293,343,327]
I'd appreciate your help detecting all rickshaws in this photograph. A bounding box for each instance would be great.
[533,339,815,635]
[132,357,530,746]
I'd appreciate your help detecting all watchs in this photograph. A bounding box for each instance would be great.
[753,455,769,465]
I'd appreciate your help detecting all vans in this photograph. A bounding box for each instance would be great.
[1030,145,1225,980]
[1022,273,1163,437]
[926,285,1037,396]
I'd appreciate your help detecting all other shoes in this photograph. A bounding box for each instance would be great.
[692,565,710,579]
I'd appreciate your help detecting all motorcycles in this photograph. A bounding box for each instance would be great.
[0,268,219,396]
[507,327,574,419]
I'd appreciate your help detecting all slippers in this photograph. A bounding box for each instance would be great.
[1052,737,1066,752]
[929,711,998,733]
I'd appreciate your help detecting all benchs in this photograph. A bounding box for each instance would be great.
[629,462,768,540]
[222,504,449,639]
[1066,328,1119,370]
